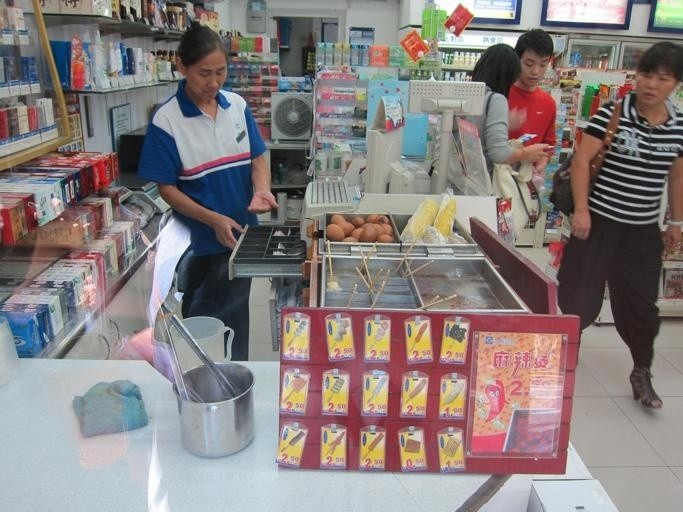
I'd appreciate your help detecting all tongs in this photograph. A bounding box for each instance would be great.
[148,290,239,405]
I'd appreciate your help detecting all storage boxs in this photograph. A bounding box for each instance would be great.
[118,123,149,174]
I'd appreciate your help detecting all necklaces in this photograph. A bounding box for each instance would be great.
[639,107,665,127]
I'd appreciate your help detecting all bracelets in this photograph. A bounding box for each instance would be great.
[666,220,682,227]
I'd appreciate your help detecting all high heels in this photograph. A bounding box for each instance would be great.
[627,365,664,410]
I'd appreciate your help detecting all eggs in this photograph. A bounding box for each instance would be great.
[326,214,395,243]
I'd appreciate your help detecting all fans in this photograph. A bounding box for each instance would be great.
[267,90,314,143]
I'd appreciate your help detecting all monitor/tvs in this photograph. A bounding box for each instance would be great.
[365,125,414,194]
[647,0,683,32]
[429,0,523,24]
[541,0,633,29]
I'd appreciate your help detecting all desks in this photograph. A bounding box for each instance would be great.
[0,354,617,512]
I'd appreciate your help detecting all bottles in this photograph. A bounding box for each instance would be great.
[674,84,683,106]
[410,47,483,82]
[572,48,609,71]
[66,0,192,92]
[579,83,636,123]
[221,29,278,138]
[621,48,647,72]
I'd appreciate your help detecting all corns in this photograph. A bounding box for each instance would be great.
[433,199,457,238]
[412,198,439,240]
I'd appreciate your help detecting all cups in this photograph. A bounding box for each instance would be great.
[167,313,235,376]
[170,362,256,460]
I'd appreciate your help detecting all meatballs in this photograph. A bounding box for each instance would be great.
[294,321,306,336]
[335,320,349,342]
[374,322,388,341]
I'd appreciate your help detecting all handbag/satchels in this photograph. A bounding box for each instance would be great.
[489,160,541,238]
[546,98,624,220]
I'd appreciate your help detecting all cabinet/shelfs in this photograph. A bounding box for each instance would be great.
[21,8,188,97]
[510,79,558,251]
[545,75,682,326]
[0,0,74,173]
[263,141,314,189]
[408,40,489,81]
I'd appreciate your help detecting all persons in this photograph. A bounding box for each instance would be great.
[137,26,280,360]
[507,29,557,196]
[194,3,206,20]
[450,43,549,198]
[557,41,683,409]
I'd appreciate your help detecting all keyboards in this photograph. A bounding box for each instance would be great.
[308,181,352,207]
